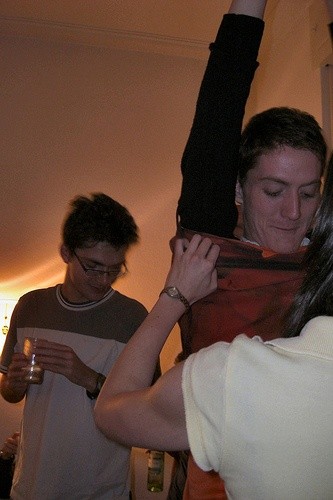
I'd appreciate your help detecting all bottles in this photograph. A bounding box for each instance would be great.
[146,449,164,493]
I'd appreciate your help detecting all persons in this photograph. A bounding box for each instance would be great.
[0,431,21,500]
[162,0,333,498]
[92,151,333,500]
[0,193,162,500]
[144,350,183,459]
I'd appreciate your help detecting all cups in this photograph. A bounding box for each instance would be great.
[19,337,44,385]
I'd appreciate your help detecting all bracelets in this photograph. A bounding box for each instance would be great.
[0,449,14,459]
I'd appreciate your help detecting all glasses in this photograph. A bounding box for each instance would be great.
[70,249,129,280]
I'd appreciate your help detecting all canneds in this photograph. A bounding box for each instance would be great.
[17,336,47,384]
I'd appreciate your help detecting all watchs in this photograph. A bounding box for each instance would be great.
[158,286,190,314]
